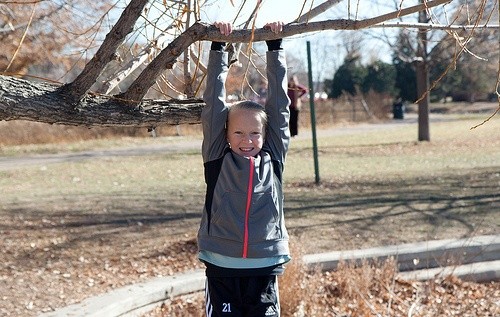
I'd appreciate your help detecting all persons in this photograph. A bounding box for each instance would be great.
[287,75,307,138]
[195,21,292,317]
[391,95,405,119]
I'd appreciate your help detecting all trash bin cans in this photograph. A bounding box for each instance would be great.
[393,102,405,119]
